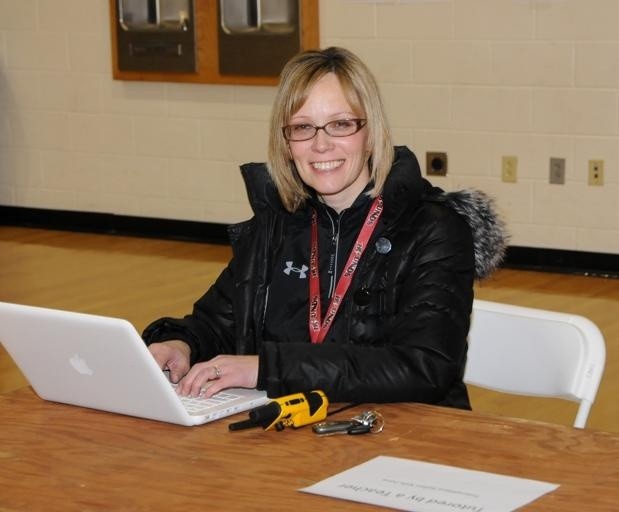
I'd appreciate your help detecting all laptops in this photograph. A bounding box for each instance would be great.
[0,301,268,426]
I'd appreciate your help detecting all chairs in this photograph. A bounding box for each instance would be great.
[459,296,607,433]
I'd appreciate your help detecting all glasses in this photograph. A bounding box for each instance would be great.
[281,118,368,142]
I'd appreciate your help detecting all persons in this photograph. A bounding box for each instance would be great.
[138,45,475,411]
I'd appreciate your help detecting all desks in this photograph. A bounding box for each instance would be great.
[0,345,619,511]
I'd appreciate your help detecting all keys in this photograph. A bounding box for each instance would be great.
[312,419,355,433]
[316,424,371,438]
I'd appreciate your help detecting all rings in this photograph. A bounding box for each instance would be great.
[213,364,222,379]
